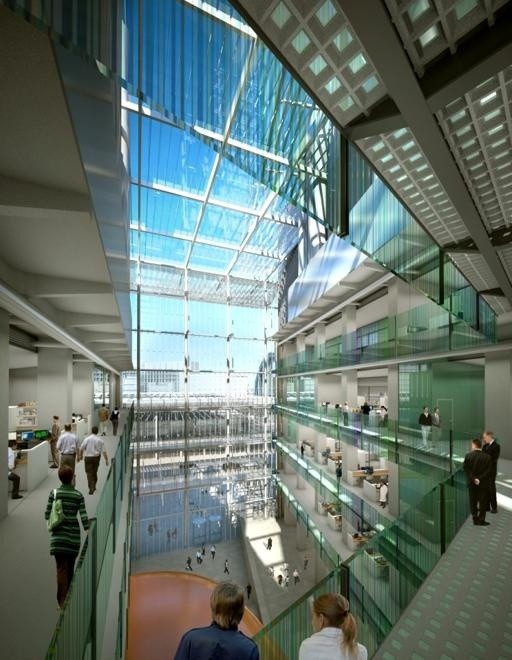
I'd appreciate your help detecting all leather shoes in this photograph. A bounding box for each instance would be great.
[12,495,23,499]
[473,507,497,525]
[89,488,96,495]
[50,464,57,468]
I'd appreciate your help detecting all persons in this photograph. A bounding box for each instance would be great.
[168,578,260,660]
[8,439,24,500]
[49,415,61,469]
[361,401,370,428]
[335,464,342,483]
[418,404,432,451]
[196,549,203,564]
[378,479,388,509]
[266,537,273,550]
[97,403,110,436]
[44,465,90,610]
[342,402,349,426]
[223,559,230,575]
[246,582,253,599]
[56,423,82,490]
[298,591,369,659]
[79,426,110,496]
[461,437,494,526]
[376,406,387,428]
[276,568,302,590]
[210,544,216,560]
[109,406,121,436]
[184,556,192,571]
[481,431,501,514]
[430,406,442,448]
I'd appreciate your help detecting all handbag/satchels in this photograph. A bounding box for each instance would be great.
[46,500,64,531]
